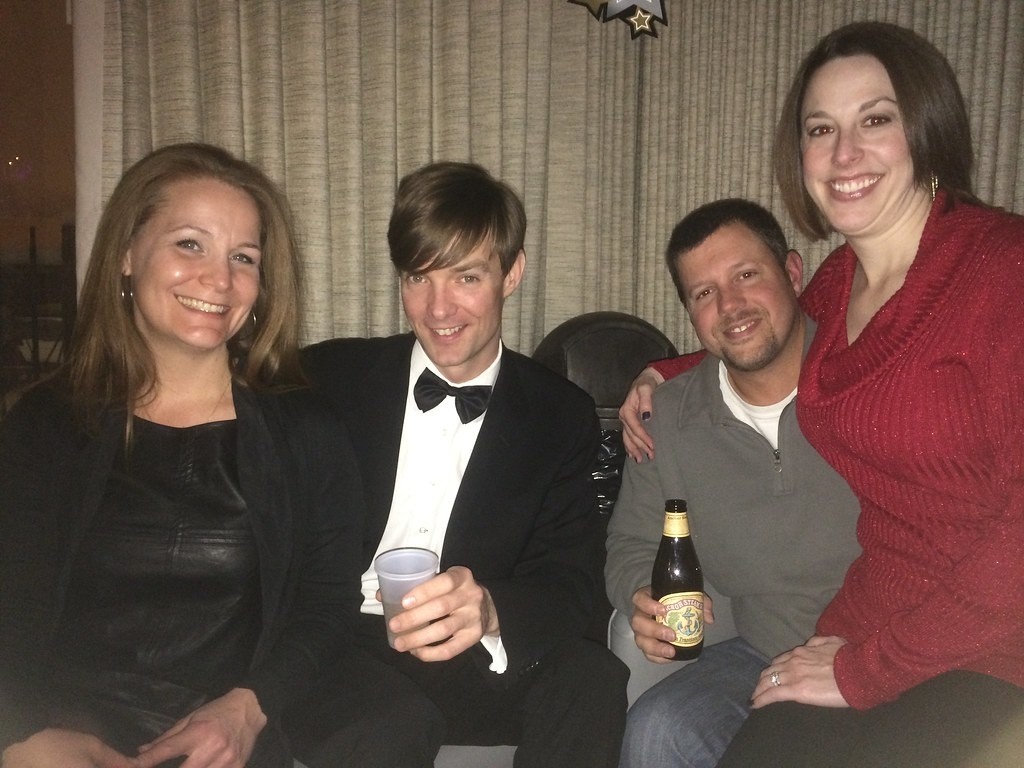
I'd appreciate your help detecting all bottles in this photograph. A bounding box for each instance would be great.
[651,499,706,661]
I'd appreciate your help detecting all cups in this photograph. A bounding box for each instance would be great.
[375,547,440,651]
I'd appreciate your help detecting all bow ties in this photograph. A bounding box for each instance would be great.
[413,367,493,424]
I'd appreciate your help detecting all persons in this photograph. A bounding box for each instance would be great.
[282,163,629,767]
[0,143,365,768]
[618,21,1024,768]
[605,198,862,768]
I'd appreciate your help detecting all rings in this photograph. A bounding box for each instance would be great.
[771,672,780,687]
